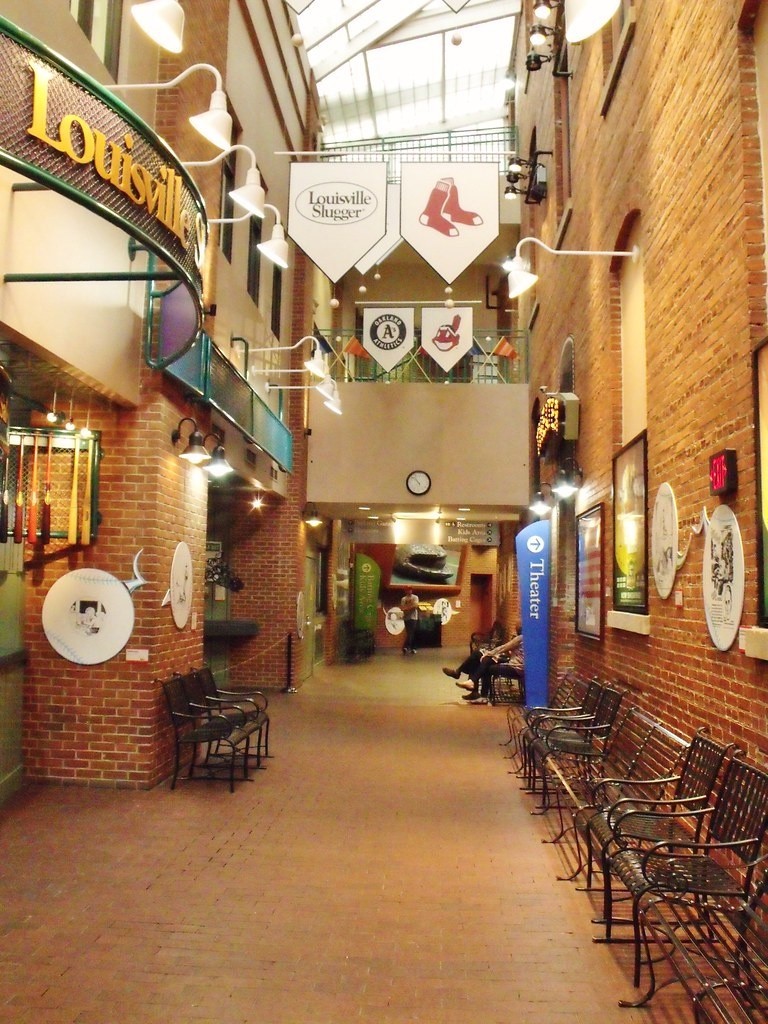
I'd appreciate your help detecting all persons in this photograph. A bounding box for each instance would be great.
[442,626,525,705]
[400,586,419,653]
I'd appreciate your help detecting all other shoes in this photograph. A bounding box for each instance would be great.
[411,649,416,654]
[462,692,481,699]
[456,683,475,690]
[471,697,489,704]
[443,668,460,679]
[402,647,407,655]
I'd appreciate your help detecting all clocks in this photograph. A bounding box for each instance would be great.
[405,470,432,496]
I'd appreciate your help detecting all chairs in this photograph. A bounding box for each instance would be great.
[154,661,276,793]
[468,621,768,988]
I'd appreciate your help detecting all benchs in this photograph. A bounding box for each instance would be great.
[613,852,768,1024]
[530,708,691,880]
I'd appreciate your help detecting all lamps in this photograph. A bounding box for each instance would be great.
[172,418,213,464]
[182,144,266,218]
[131,0,188,54]
[550,458,583,498]
[505,151,554,205]
[209,203,288,269]
[525,0,621,79]
[528,482,554,515]
[102,63,233,152]
[509,237,639,299]
[236,336,342,416]
[204,431,234,476]
[302,502,323,527]
